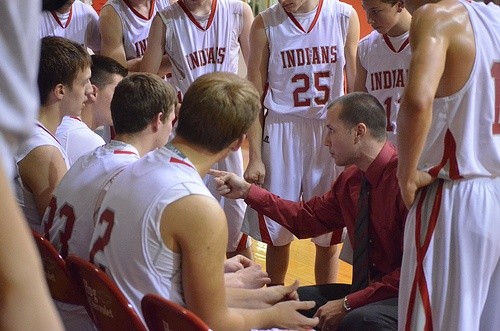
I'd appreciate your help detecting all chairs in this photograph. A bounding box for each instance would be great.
[33,231,211,331]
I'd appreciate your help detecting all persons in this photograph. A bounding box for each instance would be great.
[56,55,130,166]
[396,0,500,331]
[12,36,93,231]
[88,71,319,331]
[39,0,100,57]
[36,72,271,331]
[99,0,177,144]
[0,0,64,331]
[338,0,413,269]
[140,0,256,267]
[207,92,407,331]
[243,0,369,288]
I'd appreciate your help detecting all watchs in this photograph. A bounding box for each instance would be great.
[344,296,351,311]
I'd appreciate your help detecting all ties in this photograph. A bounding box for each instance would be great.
[350,176,369,292]
